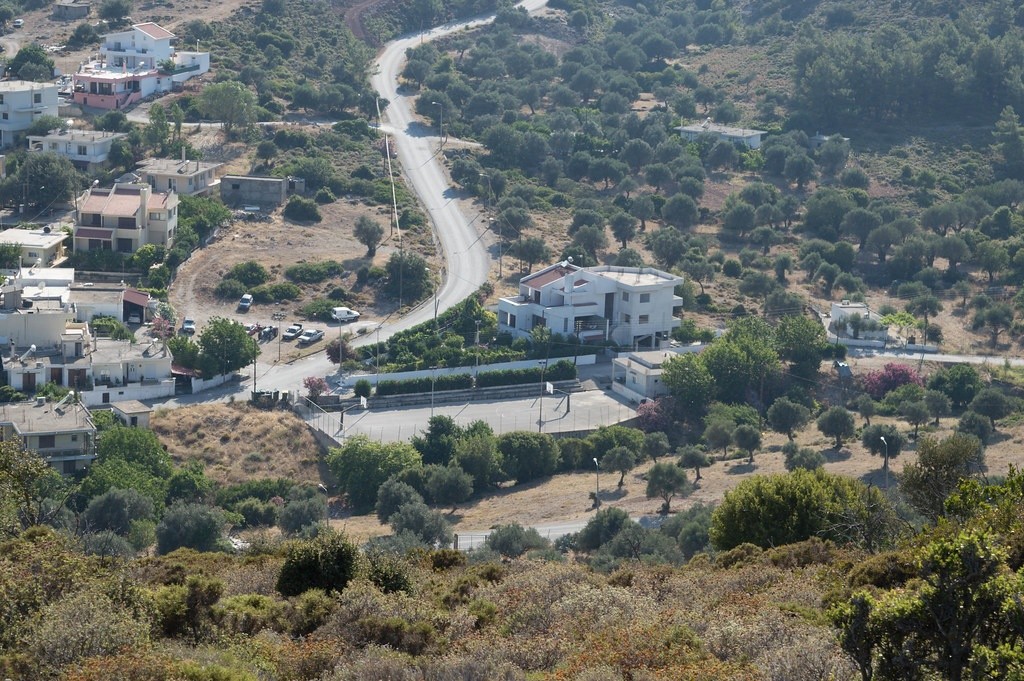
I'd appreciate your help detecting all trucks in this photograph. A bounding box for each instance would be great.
[182,316,197,333]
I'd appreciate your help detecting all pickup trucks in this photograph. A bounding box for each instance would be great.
[299,329,325,345]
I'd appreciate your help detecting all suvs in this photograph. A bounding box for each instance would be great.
[239,294,254,310]
[258,327,279,342]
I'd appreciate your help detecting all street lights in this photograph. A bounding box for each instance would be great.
[593,458,600,517]
[428,366,438,418]
[224,337,230,396]
[432,101,444,155]
[880,436,888,499]
[539,360,547,436]
[475,320,480,379]
[479,172,490,223]
[491,217,502,278]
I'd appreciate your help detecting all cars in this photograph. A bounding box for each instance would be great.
[283,323,303,341]
[245,323,260,335]
[13,17,24,28]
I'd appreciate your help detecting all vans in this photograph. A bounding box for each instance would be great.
[332,307,361,322]
